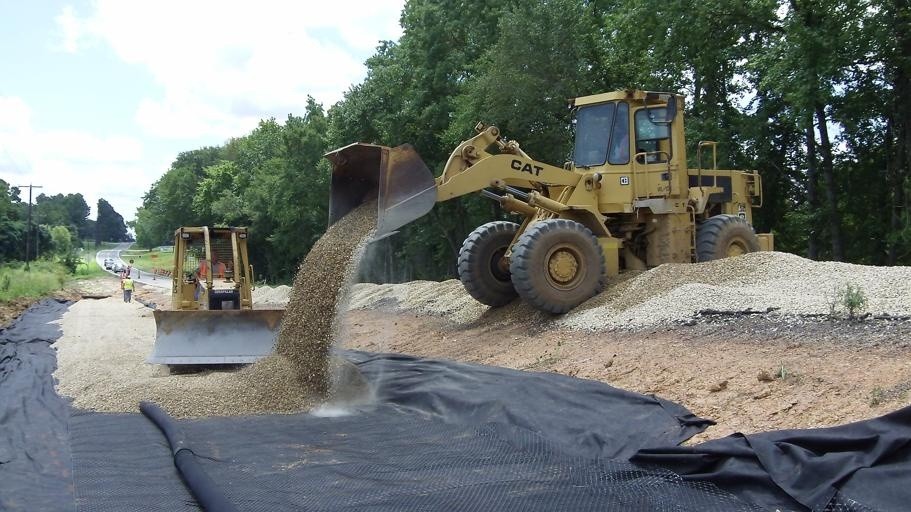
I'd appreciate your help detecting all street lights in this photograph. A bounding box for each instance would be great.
[18,185,43,270]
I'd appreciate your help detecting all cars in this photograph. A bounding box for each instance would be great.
[103,257,128,273]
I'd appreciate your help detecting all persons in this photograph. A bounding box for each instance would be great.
[194,250,228,301]
[120,268,135,303]
[612,125,630,162]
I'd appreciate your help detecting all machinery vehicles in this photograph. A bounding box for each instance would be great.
[323,84,778,314]
[143,226,288,366]
